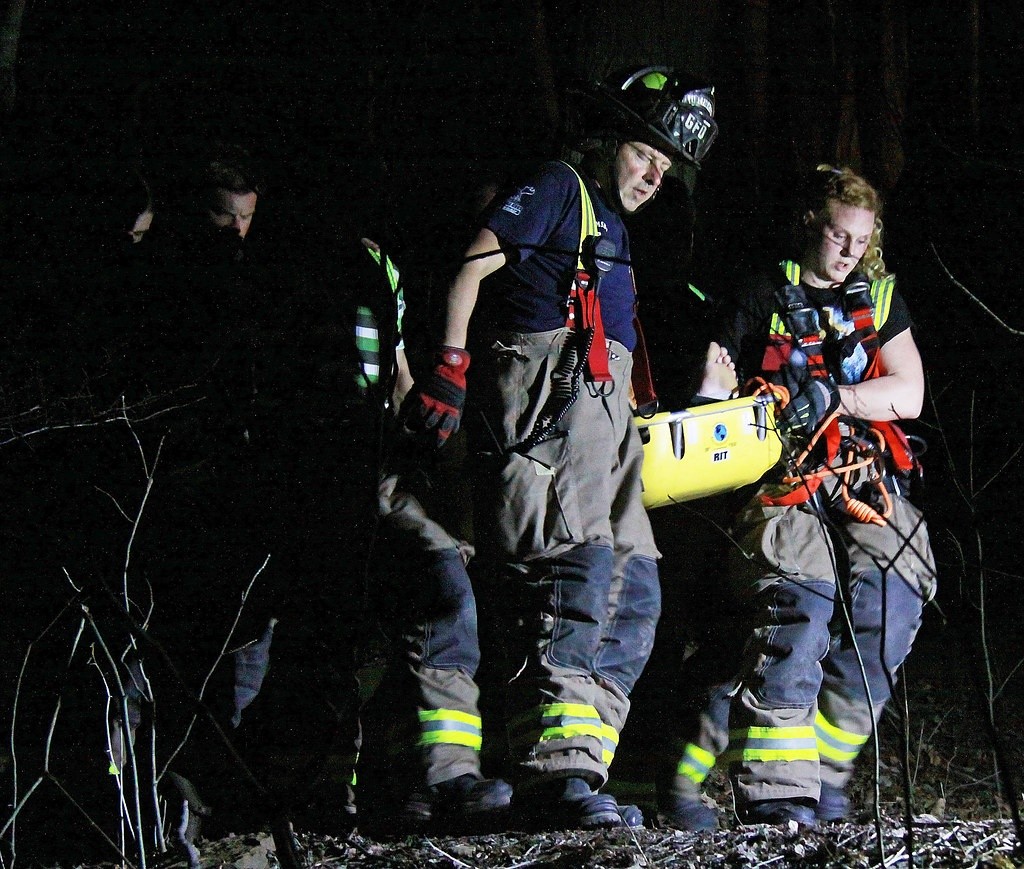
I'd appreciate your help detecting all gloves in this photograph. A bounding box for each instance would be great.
[779,372,827,431]
[409,344,470,444]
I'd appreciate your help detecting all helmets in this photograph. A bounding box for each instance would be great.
[619,66,717,168]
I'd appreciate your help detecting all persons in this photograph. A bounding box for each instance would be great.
[96,159,514,831]
[398,65,721,828]
[656,164,939,832]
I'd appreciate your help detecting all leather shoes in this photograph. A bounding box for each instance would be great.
[666,783,722,834]
[743,796,814,828]
[406,772,513,823]
[541,778,642,829]
[819,780,851,820]
